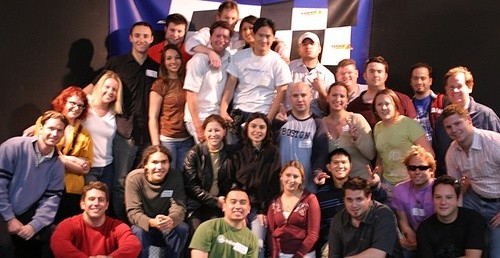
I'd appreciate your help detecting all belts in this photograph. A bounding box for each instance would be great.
[477,195,500,202]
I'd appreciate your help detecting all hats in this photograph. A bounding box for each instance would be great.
[299,32,320,44]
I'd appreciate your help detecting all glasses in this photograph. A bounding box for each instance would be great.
[407,165,429,171]
[67,100,85,109]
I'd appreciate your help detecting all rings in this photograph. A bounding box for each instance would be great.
[83,164,87,168]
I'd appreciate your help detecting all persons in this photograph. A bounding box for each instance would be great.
[328,176,398,258]
[183,0,416,239]
[107,22,162,220]
[267,160,321,258]
[34,86,94,225]
[436,66,500,178]
[391,146,439,244]
[51,182,142,258]
[188,188,258,258]
[22,71,124,217]
[124,145,189,258]
[440,104,500,258]
[148,44,194,200]
[0,111,69,258]
[407,64,448,152]
[147,14,193,72]
[415,175,490,258]
[371,89,435,203]
